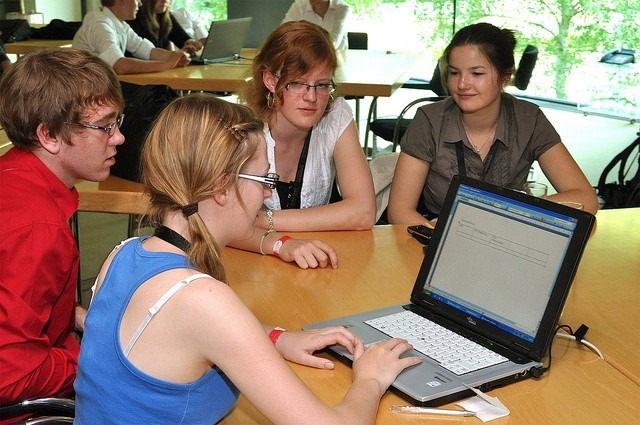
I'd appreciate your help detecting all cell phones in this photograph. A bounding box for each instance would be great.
[406,224,434,241]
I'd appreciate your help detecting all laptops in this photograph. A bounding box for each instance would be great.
[301,175,597,409]
[190,17,252,65]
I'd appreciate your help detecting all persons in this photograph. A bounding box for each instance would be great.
[388,22,598,228]
[169,0,211,47]
[0,47,125,425]
[281,0,350,55]
[72,0,191,74]
[125,0,209,57]
[74,93,422,425]
[214,19,376,268]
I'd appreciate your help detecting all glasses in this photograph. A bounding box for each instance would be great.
[78,113,124,136]
[275,73,337,95]
[239,173,280,189]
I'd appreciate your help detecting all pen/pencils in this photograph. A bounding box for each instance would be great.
[386,403,477,418]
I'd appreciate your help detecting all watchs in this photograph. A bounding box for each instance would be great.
[265,208,277,232]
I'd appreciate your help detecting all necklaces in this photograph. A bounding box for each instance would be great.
[461,118,499,154]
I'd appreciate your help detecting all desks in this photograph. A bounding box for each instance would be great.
[164,205,638,424]
[0,125,157,304]
[113,44,413,153]
[5,22,77,58]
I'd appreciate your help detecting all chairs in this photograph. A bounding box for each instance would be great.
[366,46,540,152]
[595,124,636,209]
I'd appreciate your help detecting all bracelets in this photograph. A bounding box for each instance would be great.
[268,326,287,349]
[274,231,292,259]
[259,227,275,255]
